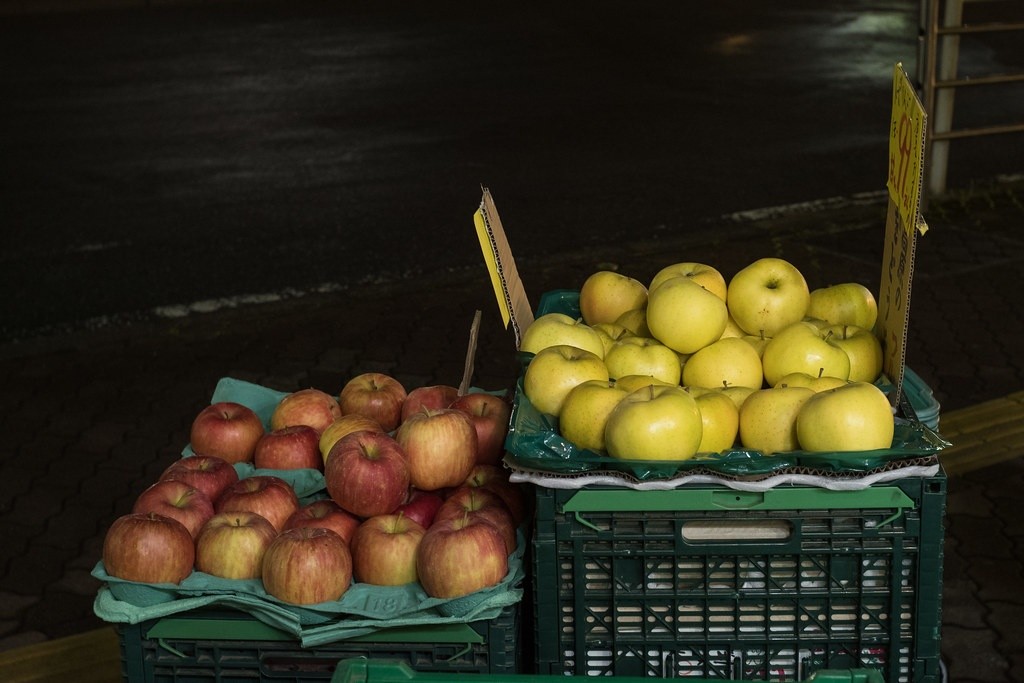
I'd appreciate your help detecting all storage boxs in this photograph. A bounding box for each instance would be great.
[500,286,949,683]
[89,388,521,683]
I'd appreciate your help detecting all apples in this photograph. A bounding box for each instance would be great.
[103,258,893,605]
